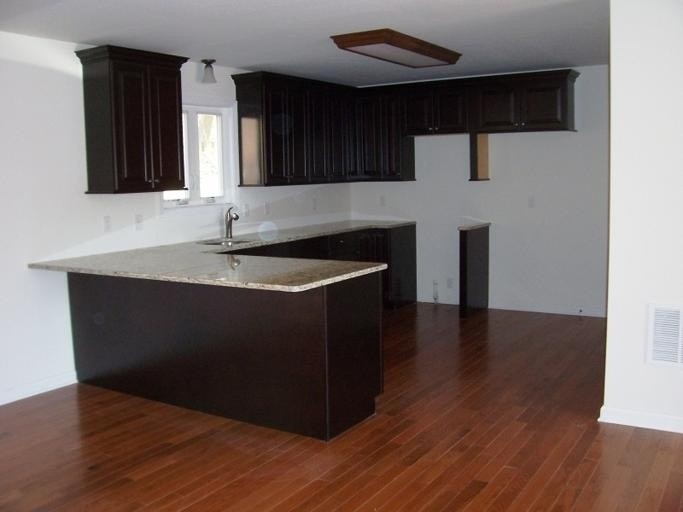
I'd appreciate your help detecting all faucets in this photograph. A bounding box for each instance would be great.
[226,254,242,272]
[224,205,240,240]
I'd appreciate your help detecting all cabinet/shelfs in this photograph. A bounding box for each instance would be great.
[362,68,581,182]
[75,44,188,194]
[360,228,390,308]
[229,71,361,187]
[285,230,361,261]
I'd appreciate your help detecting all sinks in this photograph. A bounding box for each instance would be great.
[203,239,252,246]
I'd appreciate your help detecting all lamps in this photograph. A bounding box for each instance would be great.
[330,28,462,68]
[201,59,217,84]
